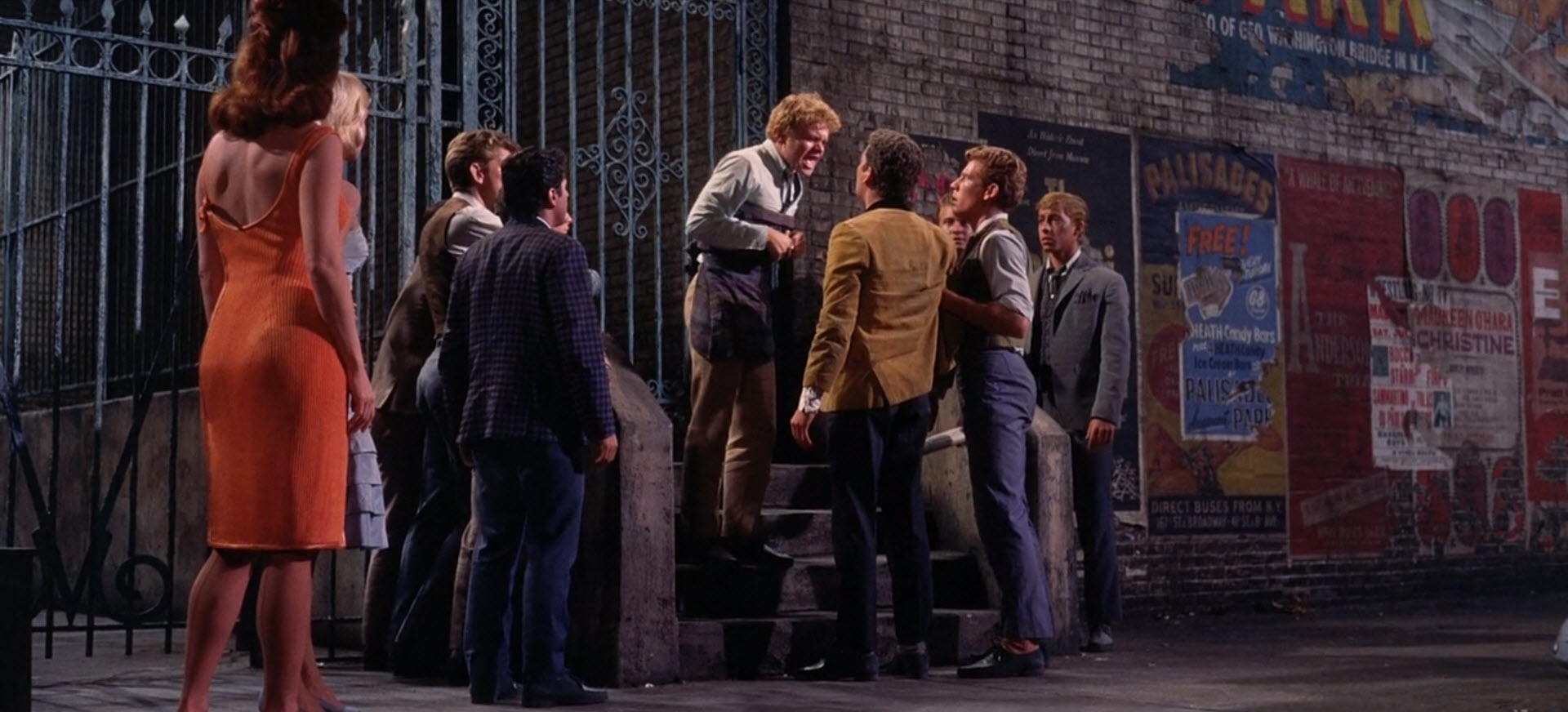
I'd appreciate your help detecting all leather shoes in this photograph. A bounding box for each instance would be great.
[879,650,930,680]
[793,651,880,680]
[969,642,1048,669]
[957,638,1045,677]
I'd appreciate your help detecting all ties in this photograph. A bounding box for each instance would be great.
[1047,266,1066,293]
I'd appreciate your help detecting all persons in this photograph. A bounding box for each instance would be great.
[442,142,619,707]
[364,131,598,684]
[933,192,976,304]
[1028,191,1126,655]
[782,127,959,684]
[677,92,845,579]
[153,0,390,712]
[940,144,1051,680]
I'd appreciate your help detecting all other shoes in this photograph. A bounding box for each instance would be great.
[522,668,609,707]
[472,679,518,705]
[1087,622,1112,651]
[678,537,723,562]
[724,541,794,569]
